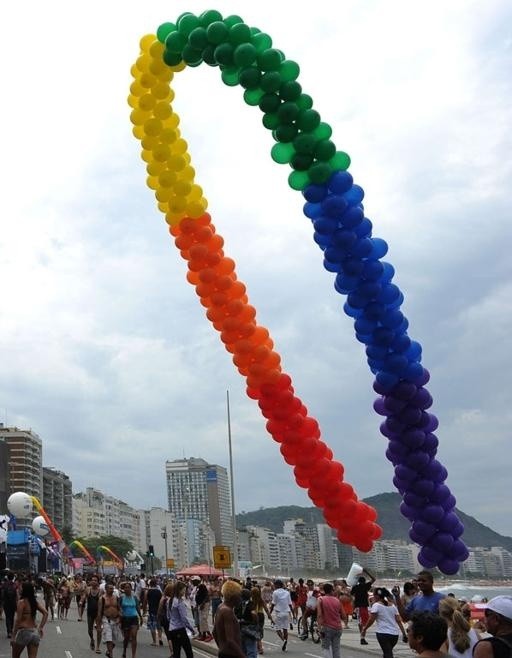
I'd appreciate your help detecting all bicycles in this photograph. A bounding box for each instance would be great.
[298,609,321,644]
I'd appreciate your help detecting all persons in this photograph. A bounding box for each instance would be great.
[2,565,511,657]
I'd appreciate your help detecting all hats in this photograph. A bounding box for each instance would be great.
[474,595,512,620]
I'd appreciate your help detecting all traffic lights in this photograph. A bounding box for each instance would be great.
[145,551,150,559]
[149,544,155,558]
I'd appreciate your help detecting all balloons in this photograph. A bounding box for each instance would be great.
[127,10,473,576]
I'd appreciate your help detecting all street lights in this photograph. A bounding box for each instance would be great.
[160,527,168,574]
